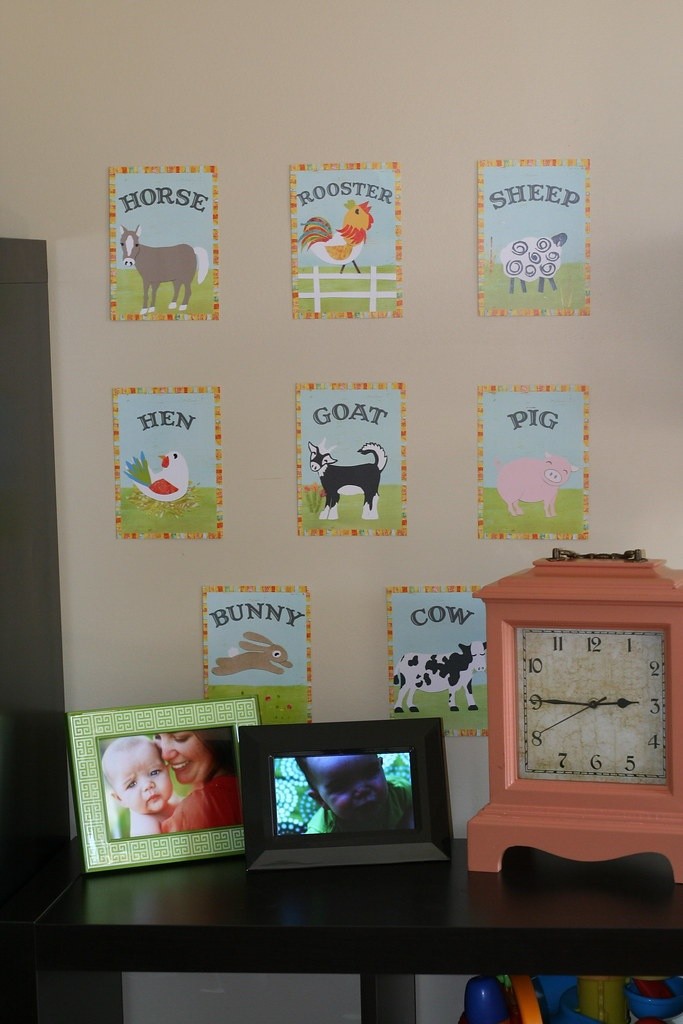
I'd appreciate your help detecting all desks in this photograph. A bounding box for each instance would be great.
[0,834,683,1024]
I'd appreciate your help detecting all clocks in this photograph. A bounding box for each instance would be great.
[464,546,683,887]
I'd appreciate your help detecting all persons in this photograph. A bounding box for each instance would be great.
[294,752,415,838]
[101,725,244,836]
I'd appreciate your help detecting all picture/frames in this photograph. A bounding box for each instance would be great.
[63,695,265,877]
[237,718,456,871]
